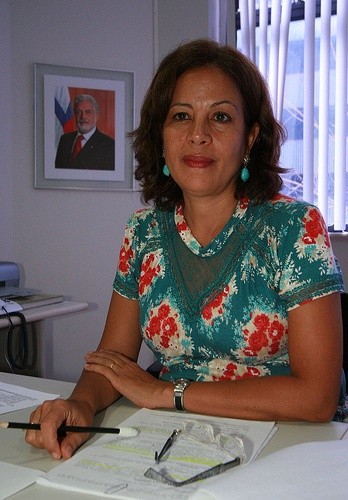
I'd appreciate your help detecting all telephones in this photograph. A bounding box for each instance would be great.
[0,298,23,314]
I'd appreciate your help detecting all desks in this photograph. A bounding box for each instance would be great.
[0,300,88,376]
[0,370,348,500]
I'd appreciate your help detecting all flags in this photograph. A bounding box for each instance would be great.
[56,85,72,154]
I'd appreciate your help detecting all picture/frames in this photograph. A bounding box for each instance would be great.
[33,62,135,192]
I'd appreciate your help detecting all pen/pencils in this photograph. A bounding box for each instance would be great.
[0,423,120,434]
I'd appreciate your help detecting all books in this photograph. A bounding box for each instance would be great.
[12,293,64,309]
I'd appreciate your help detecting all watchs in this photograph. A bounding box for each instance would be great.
[174,379,190,412]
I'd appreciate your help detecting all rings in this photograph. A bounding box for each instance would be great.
[30,422,33,424]
[111,364,114,368]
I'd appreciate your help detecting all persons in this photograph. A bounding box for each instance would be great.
[56,94,115,170]
[26,39,348,460]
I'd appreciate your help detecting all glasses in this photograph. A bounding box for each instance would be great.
[144,418,247,487]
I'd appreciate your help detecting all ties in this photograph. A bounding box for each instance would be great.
[72,135,85,158]
